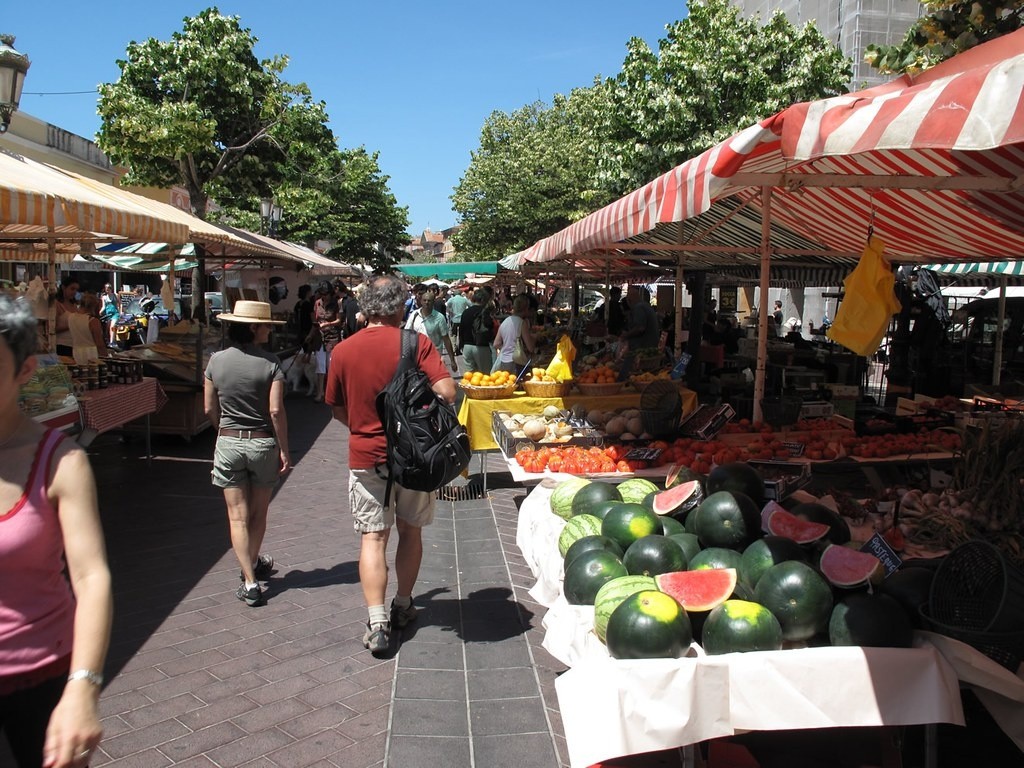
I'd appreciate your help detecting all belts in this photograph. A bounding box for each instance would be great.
[220,429,275,438]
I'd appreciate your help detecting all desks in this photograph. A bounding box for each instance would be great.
[457,385,696,495]
[504,432,967,493]
[77,377,168,462]
[516,491,1023,768]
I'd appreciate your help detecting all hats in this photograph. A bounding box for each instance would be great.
[216,300,287,324]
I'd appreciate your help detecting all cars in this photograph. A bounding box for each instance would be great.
[582,302,595,310]
[557,302,571,310]
[115,292,230,347]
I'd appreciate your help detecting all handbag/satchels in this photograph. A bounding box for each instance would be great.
[512,320,531,366]
[470,308,489,345]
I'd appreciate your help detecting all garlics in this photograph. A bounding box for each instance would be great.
[872,489,1002,538]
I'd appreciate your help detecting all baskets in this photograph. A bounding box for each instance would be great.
[633,378,682,393]
[577,382,625,396]
[457,382,519,400]
[522,379,575,397]
[641,380,683,437]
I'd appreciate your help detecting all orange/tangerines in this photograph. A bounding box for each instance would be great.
[524,367,555,382]
[459,369,517,387]
[575,366,617,384]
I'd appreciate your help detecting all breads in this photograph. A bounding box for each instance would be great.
[150,341,197,362]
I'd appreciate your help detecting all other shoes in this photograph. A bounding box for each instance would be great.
[316,392,325,401]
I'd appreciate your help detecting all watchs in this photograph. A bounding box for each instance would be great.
[66,670,105,688]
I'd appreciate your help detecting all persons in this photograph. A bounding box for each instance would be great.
[324,276,457,652]
[202,299,291,606]
[0,292,114,768]
[405,278,801,379]
[51,271,374,405]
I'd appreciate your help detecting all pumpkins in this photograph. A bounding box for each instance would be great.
[515,418,959,474]
[918,395,971,412]
[496,405,651,444]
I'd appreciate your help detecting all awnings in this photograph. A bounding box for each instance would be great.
[388,259,505,279]
[494,26,1024,424]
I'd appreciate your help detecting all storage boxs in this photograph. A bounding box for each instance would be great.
[709,726,902,768]
[492,409,602,458]
[678,403,736,441]
[799,381,1024,419]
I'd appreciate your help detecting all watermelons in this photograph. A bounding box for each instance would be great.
[549,461,913,660]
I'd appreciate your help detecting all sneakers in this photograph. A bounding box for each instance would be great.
[390,596,416,626]
[362,618,391,652]
[240,555,274,582]
[236,580,262,607]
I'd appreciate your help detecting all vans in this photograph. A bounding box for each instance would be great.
[945,296,1024,349]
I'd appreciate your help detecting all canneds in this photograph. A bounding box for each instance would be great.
[65,362,109,391]
[103,359,143,384]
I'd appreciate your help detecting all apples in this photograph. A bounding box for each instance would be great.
[578,355,604,372]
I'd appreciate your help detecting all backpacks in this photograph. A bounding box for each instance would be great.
[374,329,472,493]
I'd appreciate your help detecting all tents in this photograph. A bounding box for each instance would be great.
[0,147,356,391]
